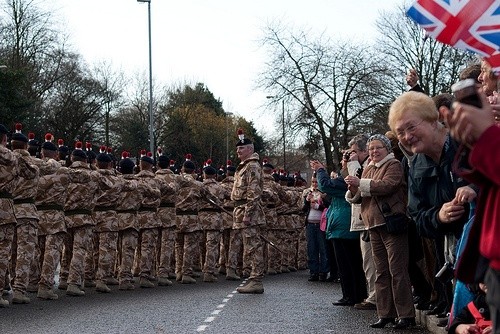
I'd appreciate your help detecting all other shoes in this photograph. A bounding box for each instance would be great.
[355,302,377,311]
[308,276,319,281]
[267,265,306,275]
[320,274,327,282]
[337,276,341,283]
[237,280,264,293]
[327,276,336,281]
[332,298,364,306]
[412,295,449,325]
[0,265,241,309]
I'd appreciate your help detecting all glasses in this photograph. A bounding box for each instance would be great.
[367,145,385,152]
[395,117,427,140]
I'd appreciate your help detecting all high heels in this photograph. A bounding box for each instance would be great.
[370,317,395,328]
[394,315,416,328]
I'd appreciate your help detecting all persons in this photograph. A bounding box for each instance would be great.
[232,128,266,294]
[0,53,500,334]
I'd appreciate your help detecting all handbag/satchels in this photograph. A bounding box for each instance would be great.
[385,214,410,235]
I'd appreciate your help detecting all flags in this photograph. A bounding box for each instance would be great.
[403,0,500,76]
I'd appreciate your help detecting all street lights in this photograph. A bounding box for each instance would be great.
[137,0,153,154]
[266,96,286,177]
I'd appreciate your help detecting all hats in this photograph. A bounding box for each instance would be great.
[0,124,236,175]
[236,128,253,147]
[262,159,306,182]
[367,134,392,147]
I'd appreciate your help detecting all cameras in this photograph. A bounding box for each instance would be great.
[343,150,352,160]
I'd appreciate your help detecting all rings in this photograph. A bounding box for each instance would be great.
[350,180,352,183]
[462,198,465,201]
[449,213,451,217]
